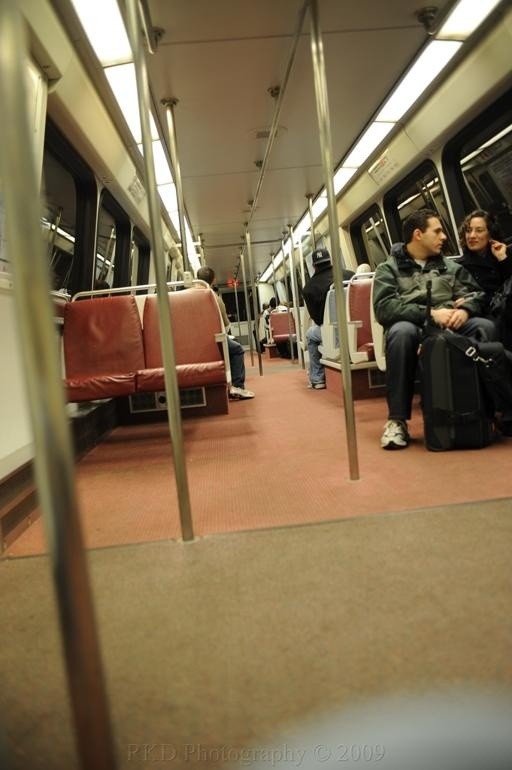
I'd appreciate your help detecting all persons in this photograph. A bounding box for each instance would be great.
[93,279,110,298]
[177,266,294,400]
[300,248,356,390]
[371,208,502,450]
[452,209,512,442]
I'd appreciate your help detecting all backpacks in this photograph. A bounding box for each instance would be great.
[419,326,491,452]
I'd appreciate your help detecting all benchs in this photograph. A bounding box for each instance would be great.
[257,255,454,404]
[58,278,230,401]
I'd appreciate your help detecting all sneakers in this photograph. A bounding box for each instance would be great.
[229,386,255,398]
[308,380,326,389]
[381,419,410,448]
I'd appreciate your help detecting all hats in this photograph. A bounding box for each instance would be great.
[312,249,331,265]
